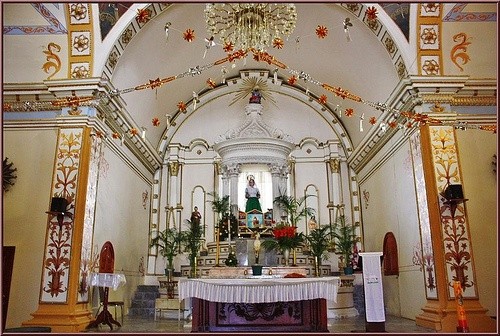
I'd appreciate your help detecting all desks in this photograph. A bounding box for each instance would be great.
[179,275,341,336]
[86,272,127,329]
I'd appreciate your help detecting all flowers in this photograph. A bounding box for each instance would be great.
[263,224,305,250]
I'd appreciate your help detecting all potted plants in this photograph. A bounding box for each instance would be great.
[330,215,358,275]
[208,192,230,268]
[300,225,340,267]
[274,186,316,254]
[177,216,205,268]
[149,211,181,275]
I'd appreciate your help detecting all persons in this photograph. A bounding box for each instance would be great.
[190,206,202,231]
[246,227,270,259]
[308,216,318,233]
[245,179,262,214]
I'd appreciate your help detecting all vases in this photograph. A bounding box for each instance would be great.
[264,246,303,268]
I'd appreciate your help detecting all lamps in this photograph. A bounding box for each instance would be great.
[199,3,299,53]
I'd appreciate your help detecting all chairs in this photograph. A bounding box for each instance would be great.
[94,282,125,323]
